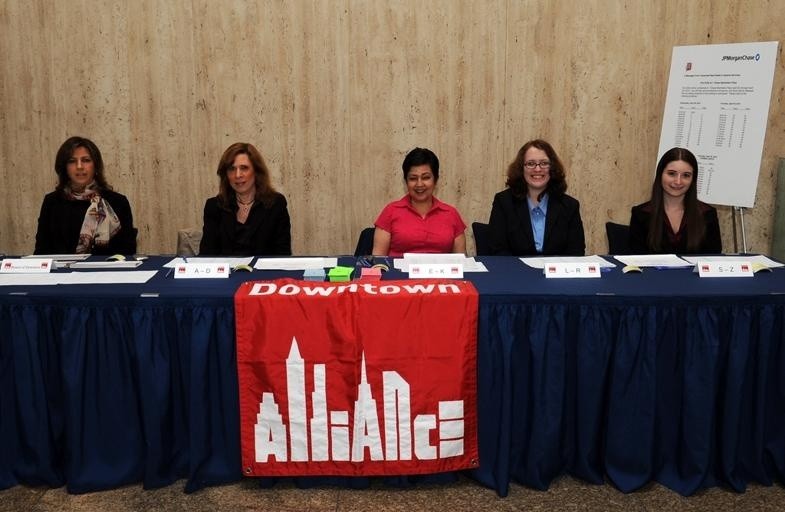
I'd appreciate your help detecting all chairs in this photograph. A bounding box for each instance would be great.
[472,221,493,255]
[132,227,138,256]
[606,221,631,255]
[354,228,375,256]
[175,230,203,257]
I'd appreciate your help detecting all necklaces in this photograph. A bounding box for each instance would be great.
[237,198,255,210]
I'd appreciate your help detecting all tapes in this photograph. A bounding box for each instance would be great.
[371,264,389,272]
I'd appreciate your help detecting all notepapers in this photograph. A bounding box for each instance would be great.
[303,268,326,282]
[359,267,382,280]
[327,266,353,282]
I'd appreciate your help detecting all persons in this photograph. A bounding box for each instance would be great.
[481,138,589,257]
[624,145,724,256]
[34,135,138,256]
[197,140,293,258]
[370,146,469,256]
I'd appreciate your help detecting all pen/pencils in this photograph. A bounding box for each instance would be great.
[359,259,371,267]
[180,255,188,264]
[384,258,391,268]
[655,266,691,270]
[165,269,174,279]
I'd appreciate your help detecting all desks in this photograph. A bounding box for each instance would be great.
[0,255,785,498]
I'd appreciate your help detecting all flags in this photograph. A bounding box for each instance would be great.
[230,277,480,479]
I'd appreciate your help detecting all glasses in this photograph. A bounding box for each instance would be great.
[522,158,553,170]
